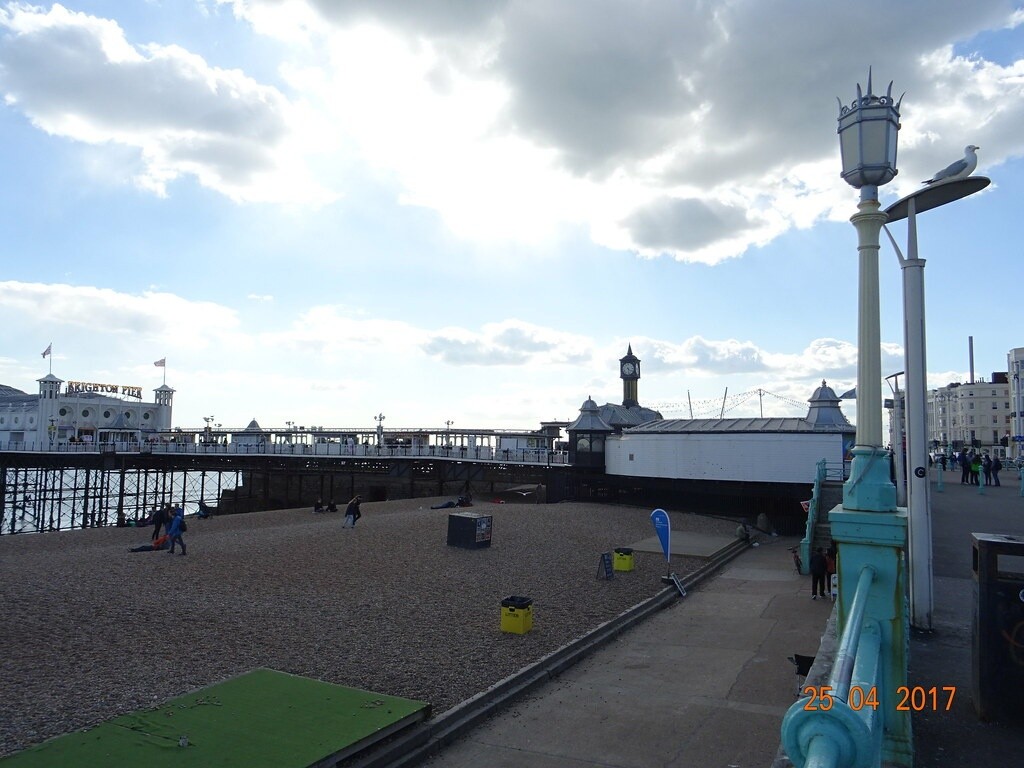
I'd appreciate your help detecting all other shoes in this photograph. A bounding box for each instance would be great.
[820,596,827,599]
[811,595,817,600]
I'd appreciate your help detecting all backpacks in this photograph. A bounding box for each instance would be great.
[179,520,187,532]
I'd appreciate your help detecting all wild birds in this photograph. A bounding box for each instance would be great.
[921,144,980,187]
[516,492,533,496]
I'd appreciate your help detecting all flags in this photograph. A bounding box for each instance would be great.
[41,343,51,358]
[153,359,165,367]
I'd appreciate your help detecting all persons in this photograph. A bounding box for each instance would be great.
[826,547,836,596]
[326,499,337,512]
[316,436,411,449]
[342,498,357,528]
[69,435,75,442]
[353,495,363,525]
[314,496,324,513]
[939,454,948,470]
[928,454,933,467]
[133,435,138,445]
[810,546,828,599]
[127,534,169,553]
[144,436,227,447]
[957,448,1003,486]
[431,491,473,509]
[167,509,187,555]
[948,452,957,471]
[736,523,750,544]
[116,500,210,541]
[77,436,84,444]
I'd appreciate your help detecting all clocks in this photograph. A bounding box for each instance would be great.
[622,363,634,376]
[635,363,640,375]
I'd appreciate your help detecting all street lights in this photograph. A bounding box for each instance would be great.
[444,419,454,458]
[203,415,215,443]
[373,413,385,458]
[286,420,296,449]
[48,414,59,449]
[832,63,915,512]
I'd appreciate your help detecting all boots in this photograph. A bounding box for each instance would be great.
[178,544,187,555]
[167,546,174,554]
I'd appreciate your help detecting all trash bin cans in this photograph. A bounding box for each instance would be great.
[500,595,534,635]
[967,531,1024,719]
[613,547,634,572]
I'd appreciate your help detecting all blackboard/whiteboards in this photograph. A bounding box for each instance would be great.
[602,553,614,578]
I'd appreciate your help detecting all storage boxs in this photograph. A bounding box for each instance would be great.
[447,511,492,550]
[613,548,634,571]
[501,596,532,634]
